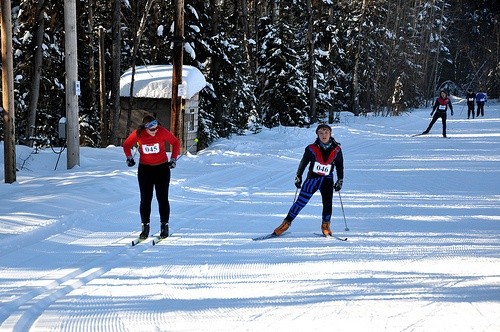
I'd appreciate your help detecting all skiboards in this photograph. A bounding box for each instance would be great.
[251,219,349,243]
[411,128,452,140]
[466,112,484,120]
[131,221,172,245]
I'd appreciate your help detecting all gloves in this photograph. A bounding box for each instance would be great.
[126,158,136,167]
[168,159,176,169]
[294,175,302,188]
[334,178,343,191]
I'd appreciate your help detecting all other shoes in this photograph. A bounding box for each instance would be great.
[443,133,446,137]
[422,130,428,134]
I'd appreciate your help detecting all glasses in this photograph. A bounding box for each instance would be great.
[148,126,158,131]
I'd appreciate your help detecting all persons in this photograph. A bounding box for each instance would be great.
[274,124,344,236]
[123,115,181,238]
[466,88,487,119]
[423,90,453,137]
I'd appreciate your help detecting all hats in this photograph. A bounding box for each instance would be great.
[316,124,332,134]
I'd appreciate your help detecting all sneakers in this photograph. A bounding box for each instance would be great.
[321,219,331,236]
[159,222,169,239]
[139,223,150,241]
[274,220,290,235]
